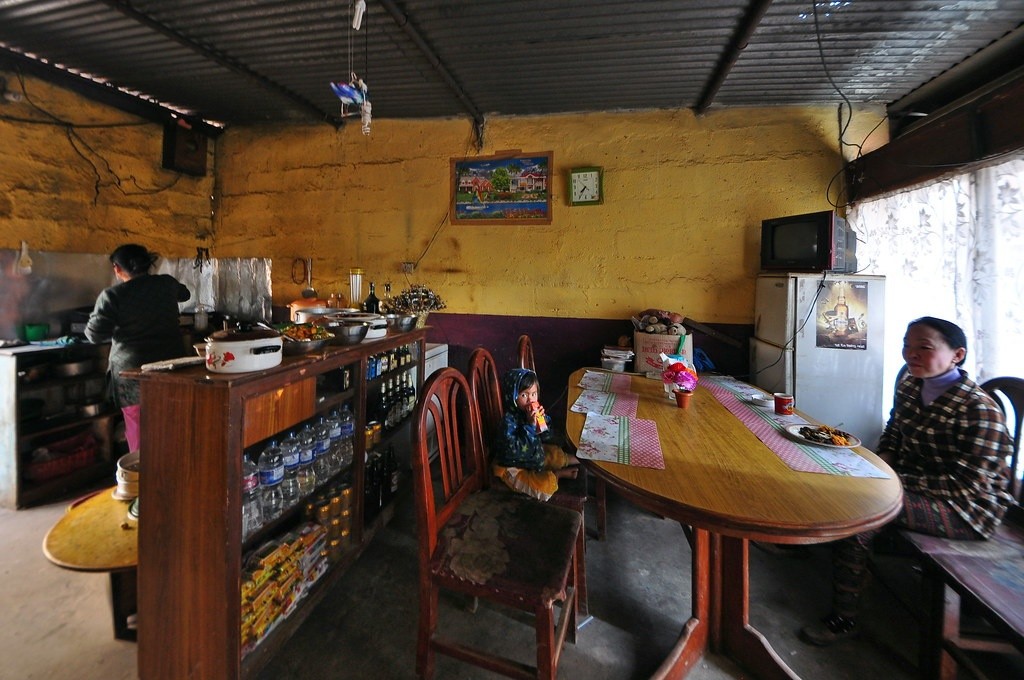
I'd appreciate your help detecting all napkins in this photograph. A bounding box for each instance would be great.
[570,389,639,419]
[577,368,631,394]
[576,410,666,471]
[696,375,891,479]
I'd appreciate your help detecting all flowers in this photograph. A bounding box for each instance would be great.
[661,362,698,392]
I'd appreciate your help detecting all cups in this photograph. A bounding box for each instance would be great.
[773,392,794,416]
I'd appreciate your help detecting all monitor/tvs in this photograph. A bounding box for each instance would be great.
[760,209,857,273]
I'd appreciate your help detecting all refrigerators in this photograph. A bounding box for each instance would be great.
[749,266,886,456]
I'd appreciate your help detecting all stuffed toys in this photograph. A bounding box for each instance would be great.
[636,309,687,336]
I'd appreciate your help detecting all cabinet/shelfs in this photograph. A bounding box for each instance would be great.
[136,327,428,680]
[1,340,118,511]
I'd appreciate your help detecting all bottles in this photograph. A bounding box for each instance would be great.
[377,281,396,314]
[363,281,380,314]
[244,345,431,556]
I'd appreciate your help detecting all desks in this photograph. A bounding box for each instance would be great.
[42,484,140,643]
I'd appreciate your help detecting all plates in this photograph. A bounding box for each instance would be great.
[784,423,861,449]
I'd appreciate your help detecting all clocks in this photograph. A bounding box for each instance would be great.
[566,164,603,207]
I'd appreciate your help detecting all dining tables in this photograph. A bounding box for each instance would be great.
[565,368,903,680]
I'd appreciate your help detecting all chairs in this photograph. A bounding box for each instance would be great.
[410,368,580,679]
[462,344,589,618]
[517,335,606,542]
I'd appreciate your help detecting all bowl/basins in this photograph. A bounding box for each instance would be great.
[81,395,110,418]
[57,358,97,376]
[189,300,418,371]
[113,449,139,500]
[26,323,49,340]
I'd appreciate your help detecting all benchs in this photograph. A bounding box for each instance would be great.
[890,376,1023,680]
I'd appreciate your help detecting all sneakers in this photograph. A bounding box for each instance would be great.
[799,610,863,646]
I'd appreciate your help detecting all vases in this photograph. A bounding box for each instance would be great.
[671,389,693,408]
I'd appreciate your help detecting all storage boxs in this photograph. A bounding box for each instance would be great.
[634,329,694,373]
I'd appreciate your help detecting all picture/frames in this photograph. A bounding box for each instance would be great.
[450,150,553,227]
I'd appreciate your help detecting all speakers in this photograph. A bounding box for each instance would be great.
[164,125,206,176]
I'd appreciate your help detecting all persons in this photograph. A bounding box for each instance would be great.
[793,317,1014,645]
[85,242,191,455]
[493,369,582,502]
